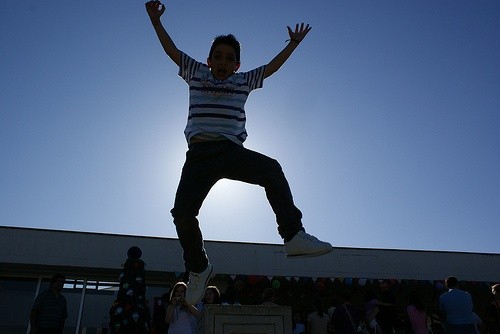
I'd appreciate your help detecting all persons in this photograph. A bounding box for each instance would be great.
[27,273,69,334]
[145,0,333,306]
[165,281,221,334]
[302,276,500,334]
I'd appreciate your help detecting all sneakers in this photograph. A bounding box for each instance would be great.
[284,230,332,259]
[186,263,214,304]
